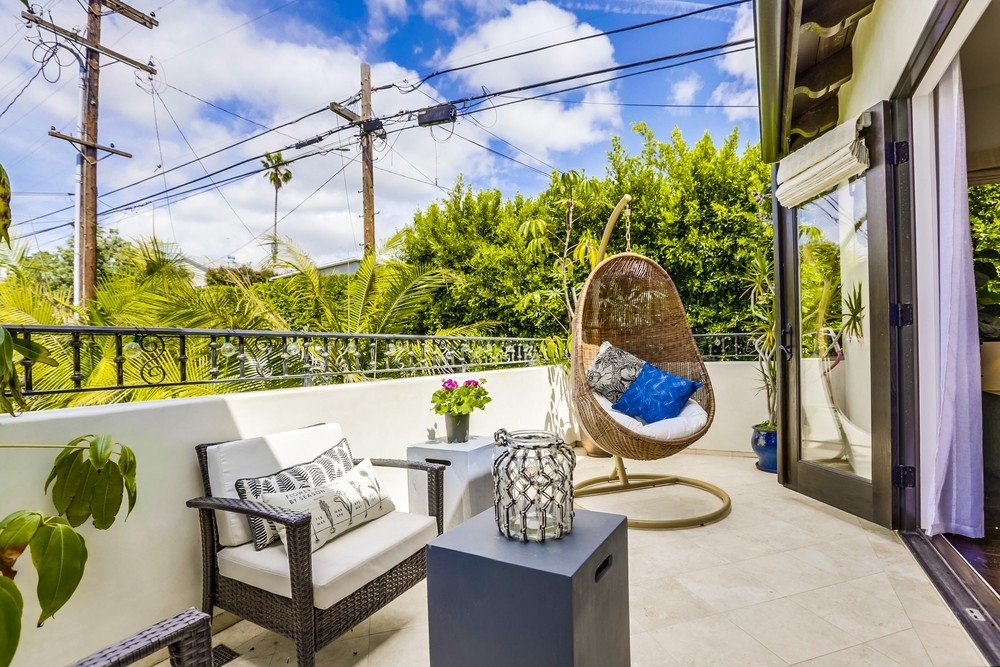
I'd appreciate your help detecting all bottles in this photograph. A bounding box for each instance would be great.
[491,429,574,541]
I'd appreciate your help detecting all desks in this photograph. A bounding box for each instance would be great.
[408,435,495,534]
[425,506,630,667]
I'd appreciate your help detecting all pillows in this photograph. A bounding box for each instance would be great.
[261,456,395,556]
[584,341,646,404]
[611,362,703,424]
[234,437,356,552]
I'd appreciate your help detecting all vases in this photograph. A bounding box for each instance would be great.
[444,412,469,443]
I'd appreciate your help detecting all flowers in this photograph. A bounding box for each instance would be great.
[429,378,491,416]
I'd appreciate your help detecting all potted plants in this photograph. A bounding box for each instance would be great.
[738,245,867,473]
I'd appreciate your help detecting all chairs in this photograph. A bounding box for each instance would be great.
[570,193,733,531]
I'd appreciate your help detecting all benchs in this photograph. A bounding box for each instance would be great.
[184,421,446,667]
